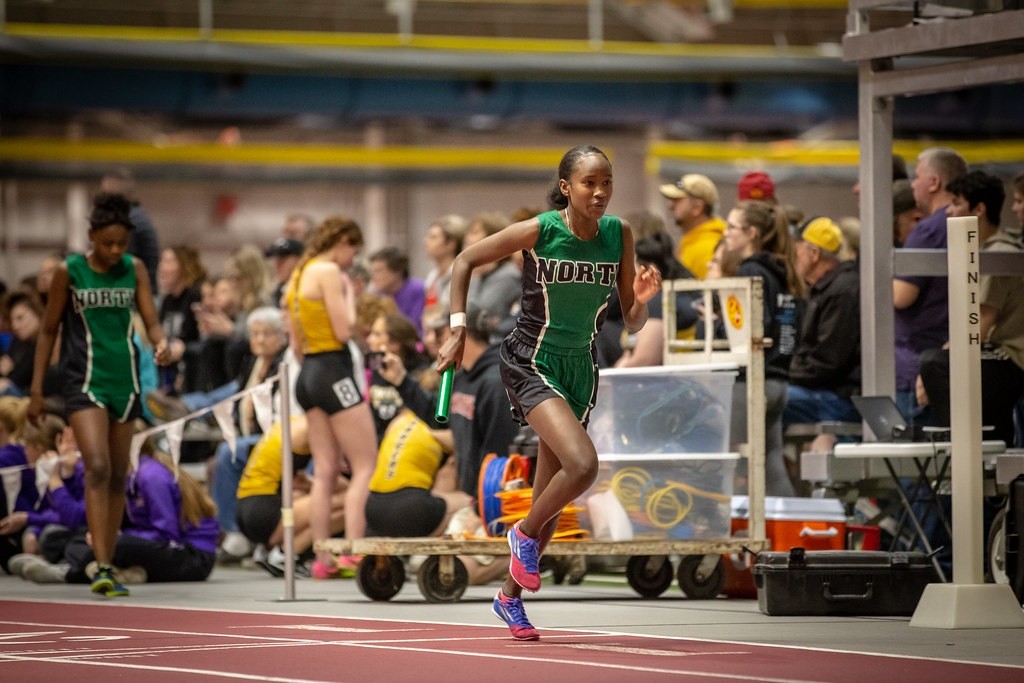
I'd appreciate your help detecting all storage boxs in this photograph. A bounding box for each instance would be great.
[575,367,847,598]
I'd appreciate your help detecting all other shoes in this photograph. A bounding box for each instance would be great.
[7,553,70,584]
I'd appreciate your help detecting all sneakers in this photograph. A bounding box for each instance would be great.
[508,518,542,593]
[87,562,131,598]
[492,588,540,642]
[255,542,360,580]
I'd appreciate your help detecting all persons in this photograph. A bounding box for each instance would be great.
[106,165,540,585]
[437,145,662,640]
[27,191,173,596]
[598,148,1024,558]
[1,254,220,583]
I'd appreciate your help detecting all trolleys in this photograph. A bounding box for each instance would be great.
[312,277,771,601]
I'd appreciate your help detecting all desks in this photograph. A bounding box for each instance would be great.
[835,440,1006,585]
[785,422,861,455]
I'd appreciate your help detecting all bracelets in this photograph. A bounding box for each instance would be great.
[451,312,467,328]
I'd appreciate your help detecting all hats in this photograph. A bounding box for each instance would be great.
[259,240,306,260]
[802,215,845,253]
[738,173,775,201]
[656,172,720,208]
